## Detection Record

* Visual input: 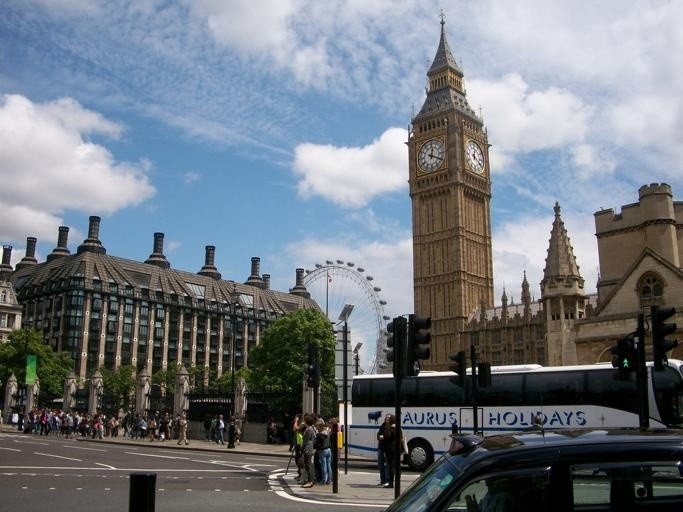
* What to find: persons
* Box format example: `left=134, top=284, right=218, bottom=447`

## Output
left=375, top=414, right=391, bottom=486
left=462, top=475, right=511, bottom=512
left=0, top=406, right=292, bottom=446
left=381, top=415, right=404, bottom=488
left=290, top=412, right=343, bottom=488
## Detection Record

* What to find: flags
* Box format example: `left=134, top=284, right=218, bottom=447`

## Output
left=24, top=355, right=37, bottom=384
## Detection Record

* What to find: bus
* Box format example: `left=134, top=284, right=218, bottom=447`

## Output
left=349, top=359, right=683, bottom=472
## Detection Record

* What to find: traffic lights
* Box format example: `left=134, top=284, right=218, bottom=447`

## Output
left=449, top=351, right=466, bottom=389
left=610, top=337, right=636, bottom=381
left=387, top=314, right=432, bottom=380
left=650, top=305, right=679, bottom=372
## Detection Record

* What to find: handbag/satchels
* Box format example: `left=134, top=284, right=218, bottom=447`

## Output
left=297, top=433, right=302, bottom=445
left=402, top=437, right=408, bottom=454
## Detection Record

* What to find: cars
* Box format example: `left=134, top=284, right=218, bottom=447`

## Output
left=383, top=428, right=683, bottom=512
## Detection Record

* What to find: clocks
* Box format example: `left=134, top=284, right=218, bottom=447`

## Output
left=416, top=138, right=445, bottom=173
left=464, top=139, right=485, bottom=175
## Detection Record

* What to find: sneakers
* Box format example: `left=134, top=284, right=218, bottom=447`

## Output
left=376, top=483, right=393, bottom=488
left=295, top=476, right=331, bottom=488
